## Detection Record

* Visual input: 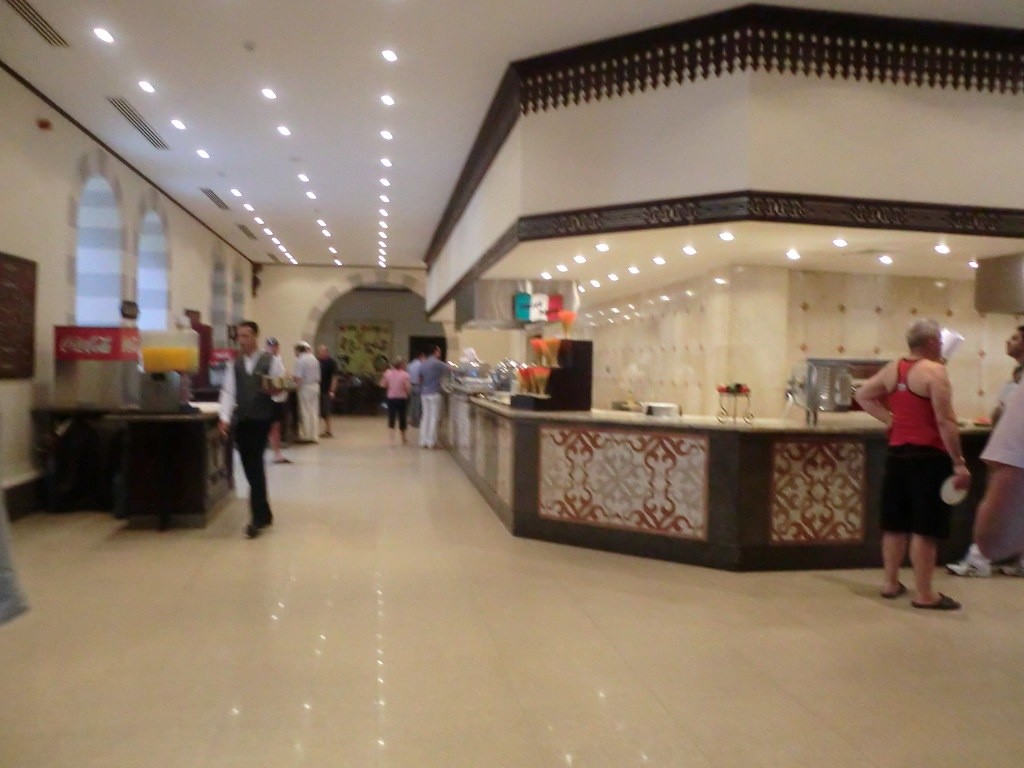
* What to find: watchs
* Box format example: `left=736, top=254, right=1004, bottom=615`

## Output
left=329, top=387, right=336, bottom=393
left=959, top=457, right=966, bottom=464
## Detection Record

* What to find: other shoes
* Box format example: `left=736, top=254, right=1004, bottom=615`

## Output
left=272, top=458, right=290, bottom=464
left=946, top=556, right=990, bottom=578
left=246, top=512, right=273, bottom=536
left=1001, top=556, right=1023, bottom=576
left=319, top=431, right=332, bottom=437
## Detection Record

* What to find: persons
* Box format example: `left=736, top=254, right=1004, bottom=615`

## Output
left=407, top=353, right=426, bottom=426
left=379, top=356, right=412, bottom=446
left=853, top=319, right=972, bottom=609
left=293, top=341, right=320, bottom=443
left=218, top=322, right=282, bottom=538
left=263, top=337, right=288, bottom=465
left=419, top=344, right=449, bottom=449
left=317, top=344, right=337, bottom=439
left=945, top=326, right=1024, bottom=577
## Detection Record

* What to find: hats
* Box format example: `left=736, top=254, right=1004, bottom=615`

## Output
left=297, top=340, right=311, bottom=349
left=940, top=328, right=966, bottom=360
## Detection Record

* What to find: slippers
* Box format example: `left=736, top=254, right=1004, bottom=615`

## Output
left=882, top=581, right=906, bottom=598
left=911, top=591, right=962, bottom=610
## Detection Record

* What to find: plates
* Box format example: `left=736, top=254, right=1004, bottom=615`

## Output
left=940, top=475, right=968, bottom=505
